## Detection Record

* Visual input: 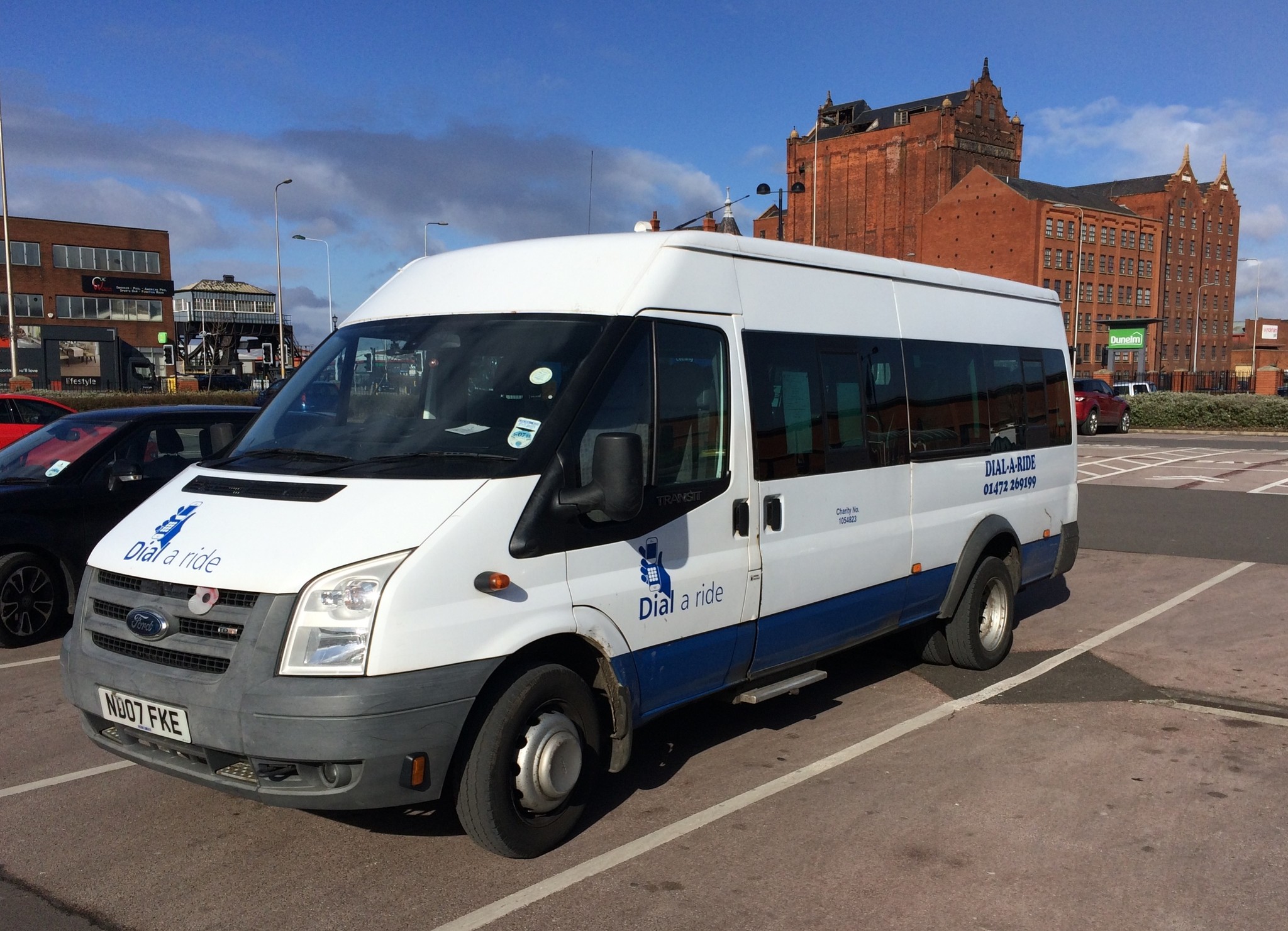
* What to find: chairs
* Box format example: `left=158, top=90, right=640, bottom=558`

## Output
left=579, top=381, right=649, bottom=487
left=59, top=350, right=69, bottom=367
left=84, top=345, right=96, bottom=364
left=144, top=426, right=193, bottom=480
left=658, top=359, right=719, bottom=482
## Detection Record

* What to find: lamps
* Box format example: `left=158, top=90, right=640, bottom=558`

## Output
left=48, top=313, right=53, bottom=318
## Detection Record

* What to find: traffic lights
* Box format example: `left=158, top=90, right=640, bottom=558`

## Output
left=262, top=343, right=273, bottom=364
left=277, top=344, right=288, bottom=364
left=163, top=344, right=175, bottom=366
left=364, top=353, right=373, bottom=373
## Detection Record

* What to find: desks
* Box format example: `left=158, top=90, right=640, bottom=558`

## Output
left=59, top=344, right=89, bottom=367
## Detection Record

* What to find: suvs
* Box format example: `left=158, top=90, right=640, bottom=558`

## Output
left=193, top=373, right=250, bottom=393
left=1113, top=381, right=1157, bottom=398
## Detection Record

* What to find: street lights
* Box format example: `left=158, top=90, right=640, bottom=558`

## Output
left=1193, top=283, right=1216, bottom=373
left=424, top=221, right=449, bottom=256
left=810, top=116, right=835, bottom=246
left=1238, top=258, right=1260, bottom=375
left=756, top=182, right=805, bottom=241
left=1052, top=203, right=1084, bottom=379
left=274, top=178, right=293, bottom=375
left=291, top=235, right=338, bottom=381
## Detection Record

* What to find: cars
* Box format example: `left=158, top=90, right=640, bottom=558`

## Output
left=1237, top=381, right=1248, bottom=391
left=1073, top=377, right=1132, bottom=436
left=0, top=392, right=160, bottom=472
left=253, top=376, right=343, bottom=414
left=362, top=377, right=395, bottom=392
left=0, top=403, right=334, bottom=649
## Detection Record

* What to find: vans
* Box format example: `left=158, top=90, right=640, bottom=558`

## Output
left=61, top=221, right=1079, bottom=860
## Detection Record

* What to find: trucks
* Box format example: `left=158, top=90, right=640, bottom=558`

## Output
left=0, top=325, right=163, bottom=392
